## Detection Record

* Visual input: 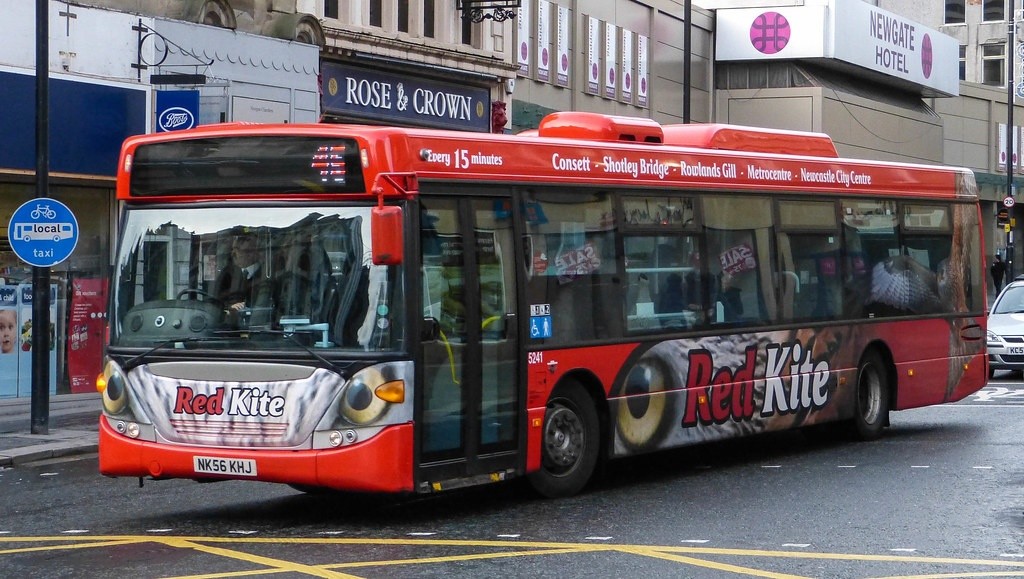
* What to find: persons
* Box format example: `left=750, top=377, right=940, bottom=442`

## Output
left=0, top=311, right=16, bottom=353
left=230, top=232, right=277, bottom=336
left=991, top=255, right=1005, bottom=298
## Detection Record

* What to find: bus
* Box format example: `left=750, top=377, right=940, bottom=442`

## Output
left=94, top=111, right=991, bottom=499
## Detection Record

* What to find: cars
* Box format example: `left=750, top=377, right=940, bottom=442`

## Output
left=987, top=274, right=1024, bottom=380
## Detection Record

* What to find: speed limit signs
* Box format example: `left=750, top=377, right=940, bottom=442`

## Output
left=1003, top=196, right=1015, bottom=208
